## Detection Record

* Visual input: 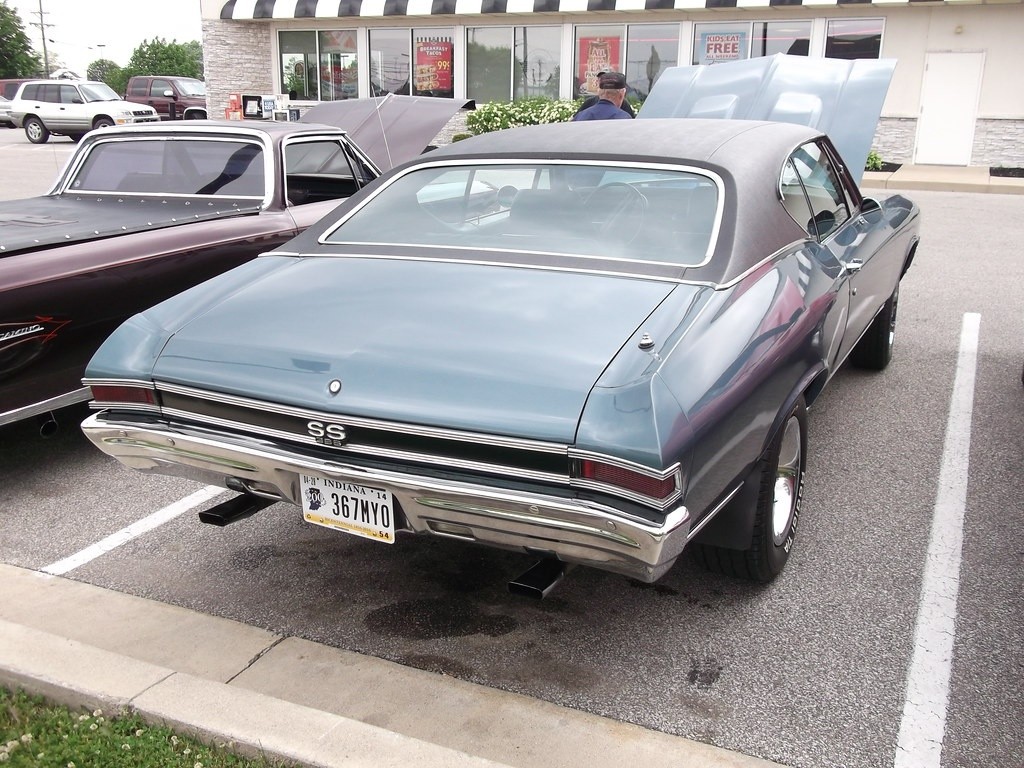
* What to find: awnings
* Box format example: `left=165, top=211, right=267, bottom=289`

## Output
left=220, top=0, right=946, bottom=19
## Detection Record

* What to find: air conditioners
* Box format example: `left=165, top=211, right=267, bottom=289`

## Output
left=273, top=109, right=300, bottom=123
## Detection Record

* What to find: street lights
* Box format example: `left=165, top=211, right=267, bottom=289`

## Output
left=96, top=44, right=106, bottom=59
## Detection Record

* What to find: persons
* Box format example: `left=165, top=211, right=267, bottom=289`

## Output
left=570, top=71, right=635, bottom=121
left=567, top=73, right=633, bottom=186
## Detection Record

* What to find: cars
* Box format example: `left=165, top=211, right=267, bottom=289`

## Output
left=0, top=92, right=496, bottom=439
left=80, top=54, right=923, bottom=606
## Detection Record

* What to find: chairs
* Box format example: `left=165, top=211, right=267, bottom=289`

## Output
left=509, top=188, right=583, bottom=234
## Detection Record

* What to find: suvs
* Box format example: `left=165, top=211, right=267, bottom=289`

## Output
left=125, top=75, right=208, bottom=120
left=6, top=78, right=161, bottom=145
left=0, top=78, right=47, bottom=129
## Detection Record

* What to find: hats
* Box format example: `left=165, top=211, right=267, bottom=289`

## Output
left=599, top=72, right=628, bottom=89
